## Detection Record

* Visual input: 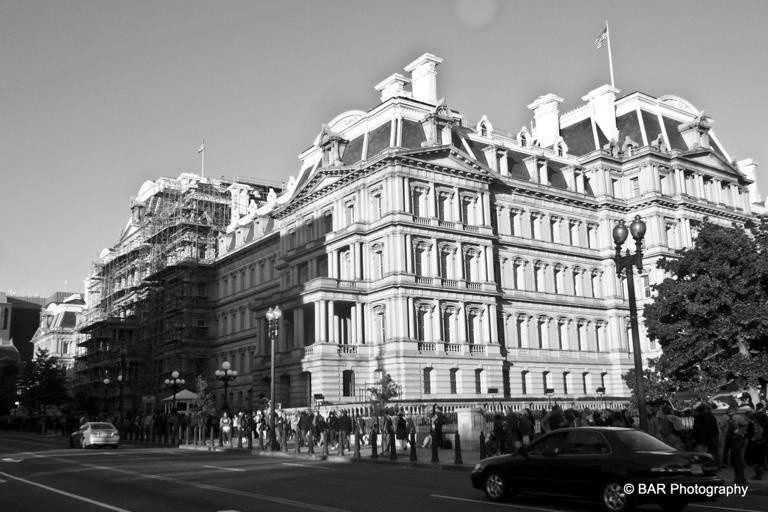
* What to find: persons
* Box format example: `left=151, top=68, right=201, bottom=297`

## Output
left=98, top=396, right=768, bottom=490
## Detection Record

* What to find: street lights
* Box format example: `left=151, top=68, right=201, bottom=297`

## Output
left=264, top=304, right=283, bottom=450
left=611, top=213, right=649, bottom=436
left=214, top=359, right=237, bottom=409
left=163, top=369, right=188, bottom=414
left=115, top=374, right=125, bottom=415
left=102, top=377, right=112, bottom=414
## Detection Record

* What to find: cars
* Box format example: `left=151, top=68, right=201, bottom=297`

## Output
left=70, top=421, right=120, bottom=449
left=467, top=425, right=725, bottom=512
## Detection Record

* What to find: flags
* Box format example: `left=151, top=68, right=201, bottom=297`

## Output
left=593, top=27, right=608, bottom=51
